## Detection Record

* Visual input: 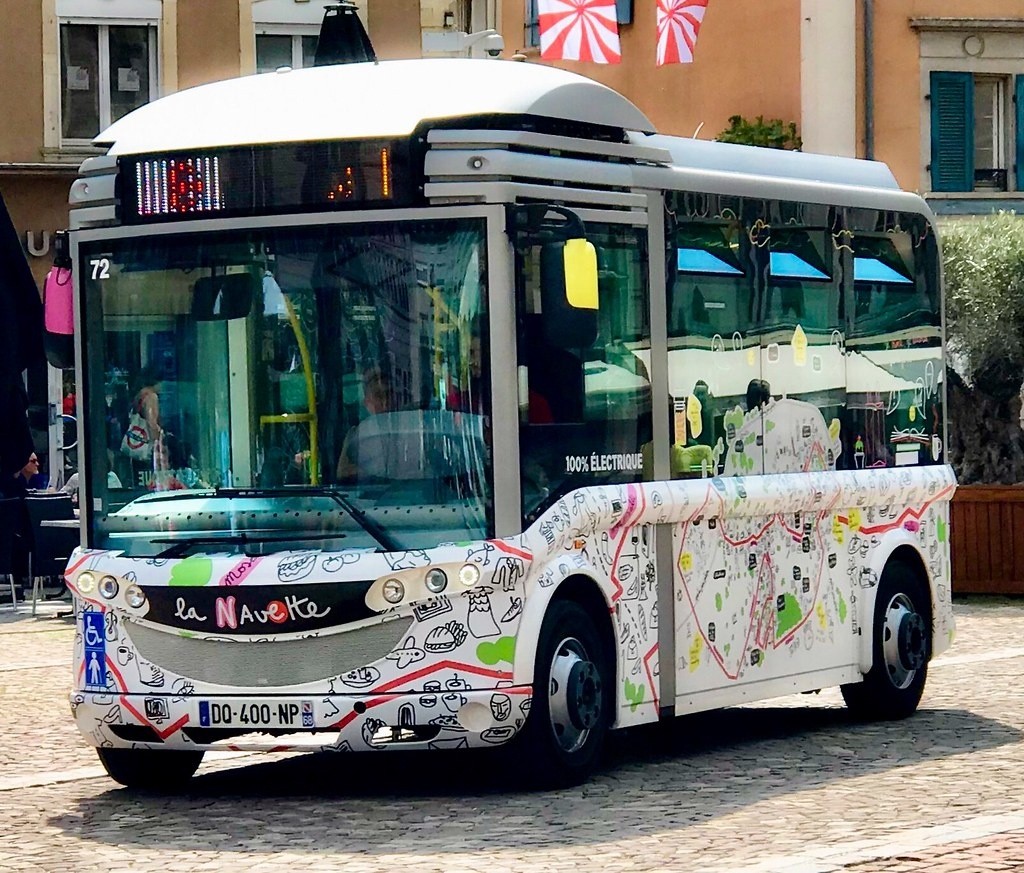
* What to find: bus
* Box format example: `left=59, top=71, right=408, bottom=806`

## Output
left=43, top=57, right=958, bottom=793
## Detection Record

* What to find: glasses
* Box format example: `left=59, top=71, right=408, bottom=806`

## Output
left=30, top=459, right=39, bottom=464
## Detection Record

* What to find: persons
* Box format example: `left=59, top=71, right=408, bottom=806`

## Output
left=128, top=368, right=165, bottom=487
left=0, top=446, right=122, bottom=503
left=358, top=366, right=387, bottom=423
left=445, top=336, right=550, bottom=424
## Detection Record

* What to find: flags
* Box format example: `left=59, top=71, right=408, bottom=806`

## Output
left=538, top=0, right=621, bottom=64
left=656, top=0, right=708, bottom=67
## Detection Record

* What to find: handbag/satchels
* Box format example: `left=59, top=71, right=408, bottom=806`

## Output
left=119, top=390, right=162, bottom=466
